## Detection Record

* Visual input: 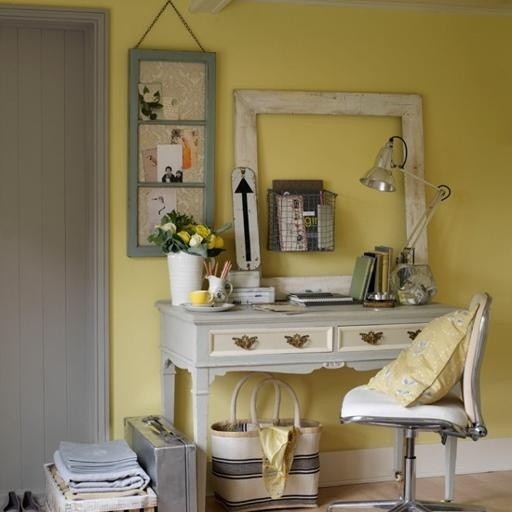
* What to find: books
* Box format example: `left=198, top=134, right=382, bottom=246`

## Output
left=289, top=245, right=394, bottom=306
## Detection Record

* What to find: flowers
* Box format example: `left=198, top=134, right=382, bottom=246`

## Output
left=147, top=206, right=226, bottom=262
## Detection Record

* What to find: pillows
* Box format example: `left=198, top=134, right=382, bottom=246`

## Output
left=367, top=306, right=480, bottom=409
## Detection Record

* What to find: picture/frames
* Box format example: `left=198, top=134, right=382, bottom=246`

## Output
left=233, top=87, right=432, bottom=303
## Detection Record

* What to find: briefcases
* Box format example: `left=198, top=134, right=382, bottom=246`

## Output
left=123, top=415, right=197, bottom=511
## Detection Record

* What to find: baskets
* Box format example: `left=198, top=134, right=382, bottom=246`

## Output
left=267, top=188, right=337, bottom=254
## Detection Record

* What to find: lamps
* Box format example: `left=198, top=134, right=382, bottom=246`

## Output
left=359, top=134, right=452, bottom=308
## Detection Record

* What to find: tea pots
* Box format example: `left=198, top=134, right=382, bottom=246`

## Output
left=205, top=274, right=233, bottom=304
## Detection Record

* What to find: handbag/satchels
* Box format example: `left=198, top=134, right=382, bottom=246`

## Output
left=210, top=373, right=322, bottom=512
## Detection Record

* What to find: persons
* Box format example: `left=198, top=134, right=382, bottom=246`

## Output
left=175, top=170, right=182, bottom=183
left=162, top=166, right=175, bottom=183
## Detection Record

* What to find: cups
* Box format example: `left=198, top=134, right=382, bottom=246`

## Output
left=188, top=289, right=214, bottom=304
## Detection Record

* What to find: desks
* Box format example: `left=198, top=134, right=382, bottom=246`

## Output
left=155, top=296, right=458, bottom=511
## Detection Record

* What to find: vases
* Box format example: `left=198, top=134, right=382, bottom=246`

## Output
left=166, top=252, right=206, bottom=306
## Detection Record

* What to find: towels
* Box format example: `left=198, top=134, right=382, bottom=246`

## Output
left=46, top=439, right=152, bottom=502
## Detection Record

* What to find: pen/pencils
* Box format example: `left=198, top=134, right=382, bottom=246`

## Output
left=203, top=261, right=233, bottom=279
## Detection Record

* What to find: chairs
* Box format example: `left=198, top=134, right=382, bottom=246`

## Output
left=328, top=291, right=493, bottom=512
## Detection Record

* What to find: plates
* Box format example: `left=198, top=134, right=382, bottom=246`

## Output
left=179, top=301, right=234, bottom=312
